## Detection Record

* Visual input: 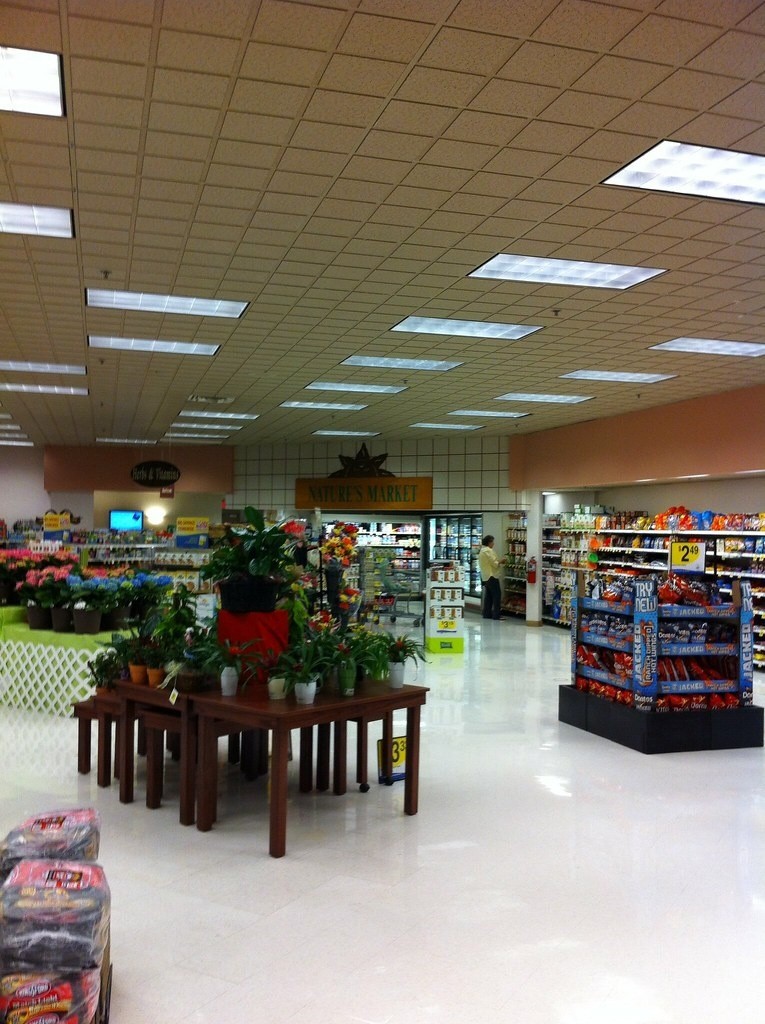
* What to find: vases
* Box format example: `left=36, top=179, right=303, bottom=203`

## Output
left=130, top=600, right=160, bottom=629
left=51, top=607, right=74, bottom=633
left=100, top=606, right=131, bottom=631
left=322, top=569, right=344, bottom=625
left=72, top=609, right=101, bottom=634
left=301, top=597, right=317, bottom=616
left=27, top=606, right=52, bottom=630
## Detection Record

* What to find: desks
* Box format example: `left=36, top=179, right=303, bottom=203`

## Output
left=113, top=677, right=329, bottom=824
left=191, top=682, right=431, bottom=859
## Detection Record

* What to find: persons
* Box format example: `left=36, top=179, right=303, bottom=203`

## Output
left=478, top=535, right=508, bottom=620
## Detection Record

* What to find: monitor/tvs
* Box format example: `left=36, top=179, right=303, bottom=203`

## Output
left=108, top=510, right=144, bottom=533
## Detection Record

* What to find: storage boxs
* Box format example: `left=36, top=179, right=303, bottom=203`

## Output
left=186, top=549, right=199, bottom=568
left=430, top=589, right=441, bottom=600
left=171, top=550, right=182, bottom=565
left=199, top=572, right=210, bottom=591
left=177, top=549, right=187, bottom=565
left=186, top=572, right=199, bottom=592
left=452, top=589, right=462, bottom=599
left=175, top=571, right=186, bottom=591
left=162, top=551, right=171, bottom=564
left=452, top=608, right=462, bottom=618
left=199, top=549, right=213, bottom=565
left=455, top=571, right=463, bottom=581
left=442, top=607, right=452, bottom=618
left=431, top=571, right=444, bottom=581
left=154, top=552, right=164, bottom=563
left=431, top=607, right=442, bottom=617
left=174, top=517, right=208, bottom=550
left=444, top=571, right=455, bottom=581
left=441, top=589, right=452, bottom=599
left=43, top=514, right=71, bottom=543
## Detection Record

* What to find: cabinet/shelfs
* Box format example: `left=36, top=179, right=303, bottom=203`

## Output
left=542, top=503, right=765, bottom=673
left=501, top=514, right=527, bottom=617
left=0, top=529, right=217, bottom=621
left=425, top=559, right=464, bottom=654
left=559, top=569, right=765, bottom=756
left=345, top=520, right=422, bottom=594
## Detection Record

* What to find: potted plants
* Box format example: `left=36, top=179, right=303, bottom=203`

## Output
left=381, top=631, right=429, bottom=689
left=282, top=641, right=332, bottom=705
left=254, top=650, right=291, bottom=700
left=88, top=656, right=115, bottom=697
left=143, top=641, right=172, bottom=687
left=328, top=630, right=376, bottom=697
left=199, top=639, right=264, bottom=698
left=197, top=506, right=304, bottom=682
left=332, top=635, right=378, bottom=687
left=123, top=636, right=147, bottom=685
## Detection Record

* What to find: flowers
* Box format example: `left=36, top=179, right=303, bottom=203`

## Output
left=229, top=647, right=243, bottom=668
left=133, top=573, right=175, bottom=600
left=15, top=569, right=56, bottom=607
left=37, top=567, right=74, bottom=609
left=317, top=522, right=359, bottom=571
left=395, top=640, right=407, bottom=650
left=337, top=584, right=363, bottom=626
left=301, top=571, right=321, bottom=599
left=0, top=547, right=82, bottom=580
left=66, top=575, right=114, bottom=613
left=307, top=611, right=341, bottom=638
left=101, top=572, right=142, bottom=607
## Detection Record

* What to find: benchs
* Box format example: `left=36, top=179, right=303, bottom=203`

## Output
left=143, top=709, right=276, bottom=827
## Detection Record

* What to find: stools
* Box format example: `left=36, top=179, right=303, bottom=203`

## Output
left=73, top=700, right=146, bottom=786
left=346, top=710, right=393, bottom=793
